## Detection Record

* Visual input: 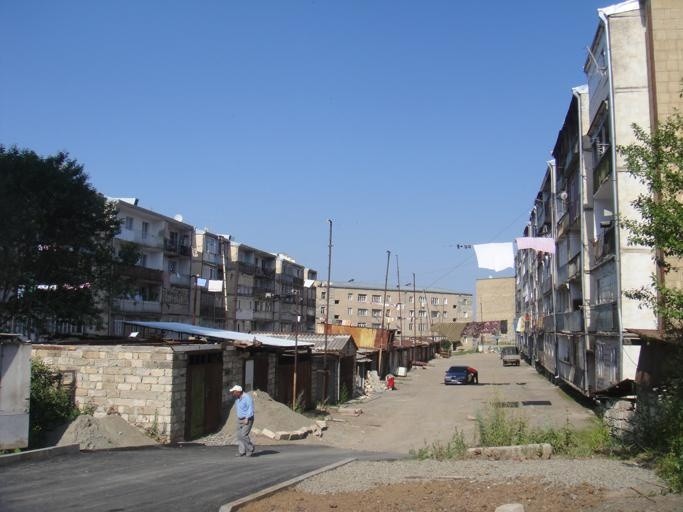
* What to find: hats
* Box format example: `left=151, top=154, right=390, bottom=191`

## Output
left=229, top=385, right=242, bottom=392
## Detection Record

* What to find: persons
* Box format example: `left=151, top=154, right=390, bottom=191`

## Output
left=229, top=385, right=255, bottom=456
left=386, top=373, right=394, bottom=390
left=467, top=367, right=478, bottom=384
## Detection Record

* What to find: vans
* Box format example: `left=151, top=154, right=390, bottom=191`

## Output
left=501, top=345, right=521, bottom=366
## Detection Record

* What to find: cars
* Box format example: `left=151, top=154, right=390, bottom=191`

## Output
left=444, top=366, right=478, bottom=385
